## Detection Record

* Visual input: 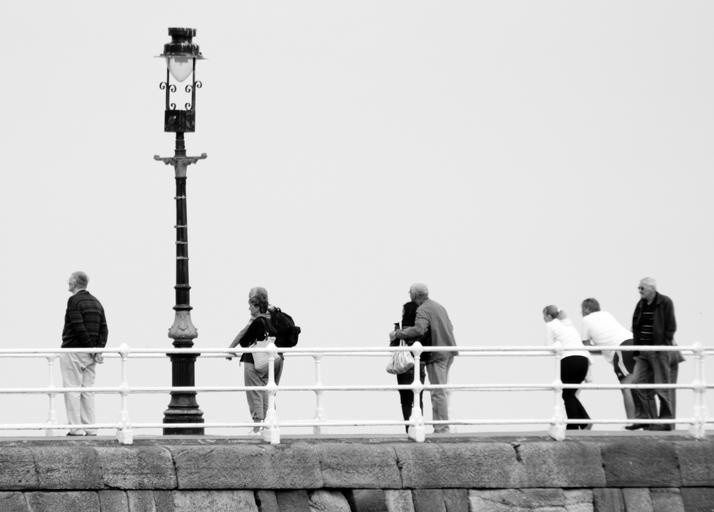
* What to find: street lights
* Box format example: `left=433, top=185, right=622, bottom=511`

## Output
left=150, top=24, right=214, bottom=439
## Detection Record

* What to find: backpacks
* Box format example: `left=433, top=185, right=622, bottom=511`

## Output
left=266, top=306, right=301, bottom=348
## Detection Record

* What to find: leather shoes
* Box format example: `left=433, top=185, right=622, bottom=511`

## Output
left=624, top=422, right=650, bottom=431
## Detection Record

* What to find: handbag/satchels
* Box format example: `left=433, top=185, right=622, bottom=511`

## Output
left=248, top=335, right=282, bottom=373
left=668, top=344, right=686, bottom=367
left=385, top=337, right=415, bottom=375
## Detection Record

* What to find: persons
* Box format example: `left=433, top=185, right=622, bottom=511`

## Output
left=58, top=271, right=108, bottom=437
left=240, top=292, right=273, bottom=434
left=625, top=277, right=684, bottom=431
left=580, top=299, right=644, bottom=430
left=390, top=283, right=459, bottom=435
left=226, top=288, right=285, bottom=387
left=542, top=306, right=593, bottom=430
